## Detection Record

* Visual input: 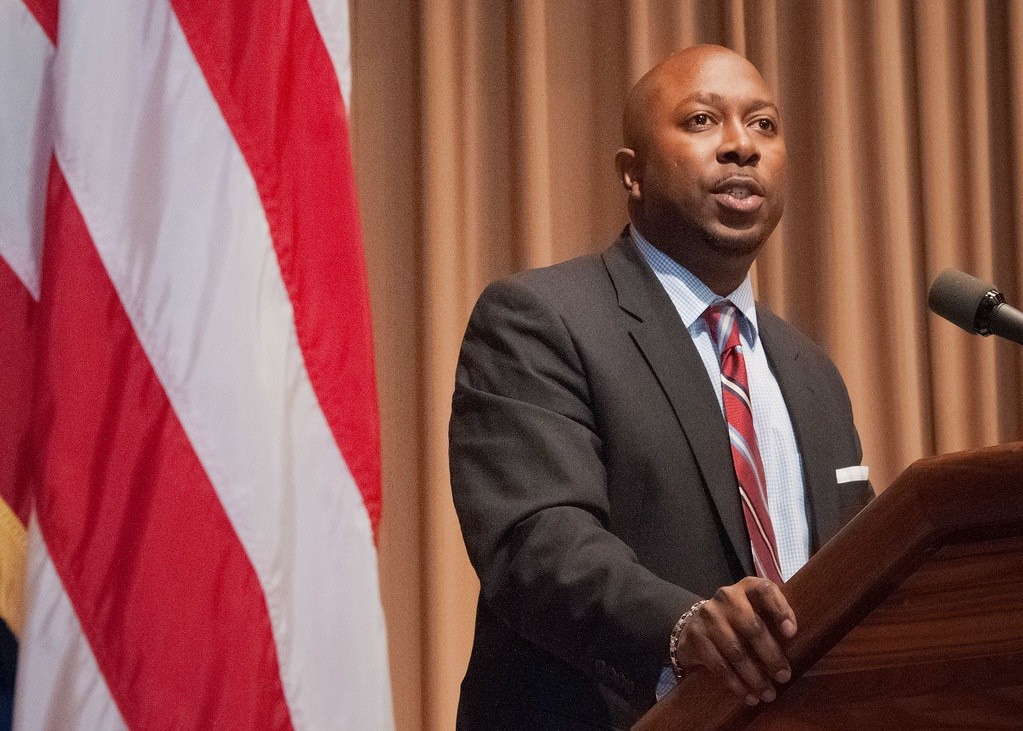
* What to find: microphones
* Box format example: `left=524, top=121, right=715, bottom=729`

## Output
left=928, top=268, right=1023, bottom=344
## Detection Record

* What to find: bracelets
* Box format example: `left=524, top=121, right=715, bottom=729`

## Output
left=670, top=600, right=709, bottom=682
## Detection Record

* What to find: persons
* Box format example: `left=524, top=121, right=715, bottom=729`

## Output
left=449, top=45, right=877, bottom=731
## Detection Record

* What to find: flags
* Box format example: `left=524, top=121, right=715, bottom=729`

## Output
left=1, top=2, right=395, bottom=730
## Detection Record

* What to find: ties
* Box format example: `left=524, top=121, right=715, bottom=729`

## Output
left=699, top=299, right=784, bottom=591
